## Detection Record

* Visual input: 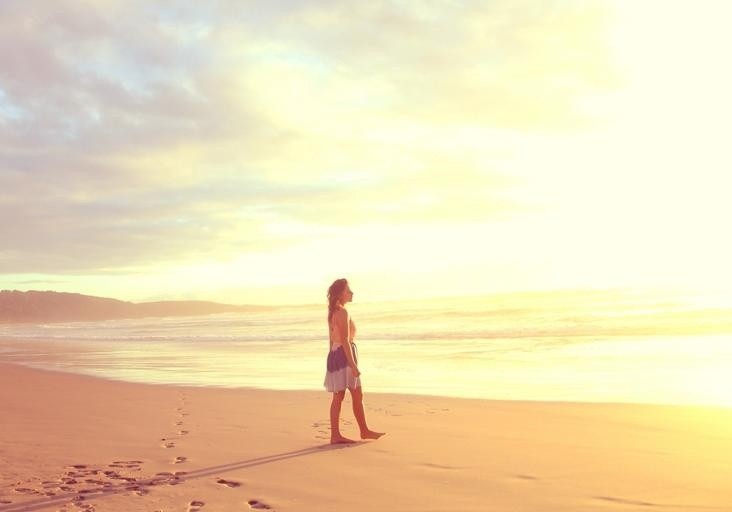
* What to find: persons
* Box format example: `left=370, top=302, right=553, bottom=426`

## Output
left=322, top=277, right=386, bottom=444
